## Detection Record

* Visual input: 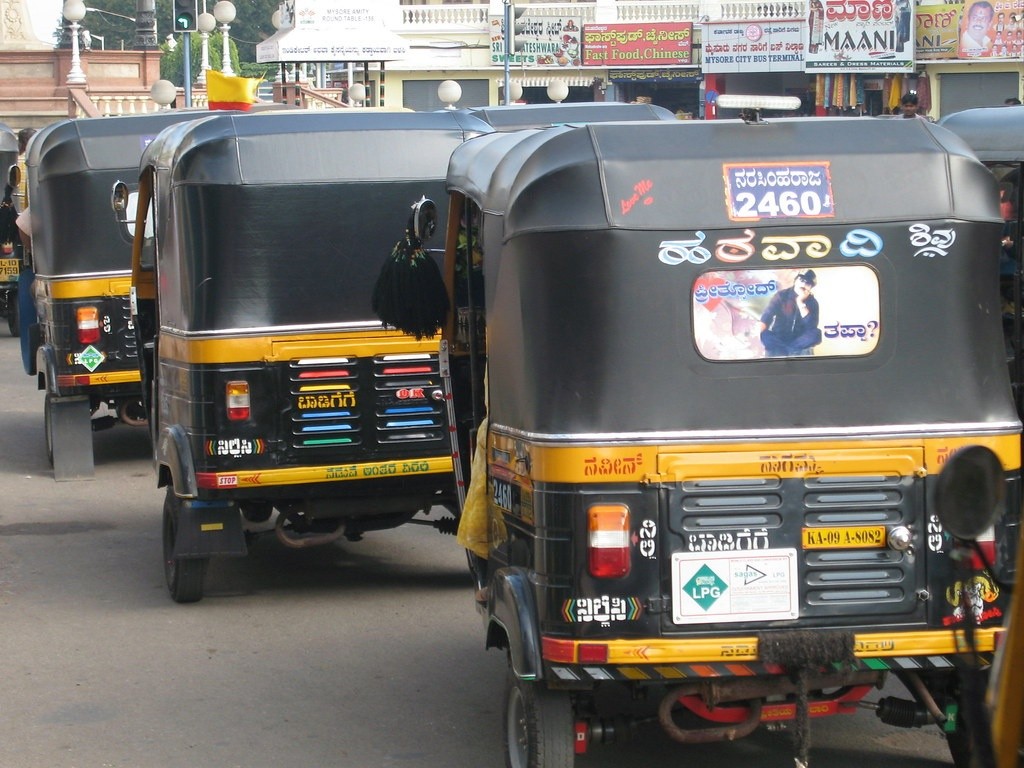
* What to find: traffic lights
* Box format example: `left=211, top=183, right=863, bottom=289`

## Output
left=173, top=0, right=199, bottom=33
left=509, top=4, right=527, bottom=56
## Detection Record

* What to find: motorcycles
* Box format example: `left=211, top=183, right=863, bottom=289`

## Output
left=452, top=102, right=680, bottom=132
left=110, top=111, right=498, bottom=603
left=407, top=94, right=1022, bottom=767
left=934, top=444, right=1024, bottom=768
left=933, top=106, right=1023, bottom=409
left=6, top=110, right=250, bottom=482
left=0, top=127, right=26, bottom=332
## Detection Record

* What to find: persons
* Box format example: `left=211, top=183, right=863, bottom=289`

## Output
left=15, top=205, right=34, bottom=253
left=897, top=92, right=929, bottom=122
left=751, top=270, right=825, bottom=359
left=960, top=1, right=993, bottom=55
left=990, top=11, right=1024, bottom=58
left=1005, top=97, right=1022, bottom=106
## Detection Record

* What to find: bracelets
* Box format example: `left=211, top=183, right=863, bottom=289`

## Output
left=798, top=303, right=807, bottom=309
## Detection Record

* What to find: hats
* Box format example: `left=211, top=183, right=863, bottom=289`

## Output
left=798, top=269, right=817, bottom=285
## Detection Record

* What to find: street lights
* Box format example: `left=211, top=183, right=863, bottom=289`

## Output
left=214, top=1, right=236, bottom=76
left=151, top=79, right=176, bottom=112
left=437, top=80, right=462, bottom=110
left=197, top=13, right=216, bottom=83
left=350, top=83, right=365, bottom=107
left=62, top=0, right=88, bottom=119
left=271, top=10, right=294, bottom=83
left=85, top=7, right=158, bottom=43
left=82, top=30, right=104, bottom=51
left=503, top=82, right=523, bottom=103
left=547, top=80, right=568, bottom=103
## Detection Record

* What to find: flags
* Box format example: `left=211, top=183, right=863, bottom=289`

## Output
left=204, top=68, right=261, bottom=112
